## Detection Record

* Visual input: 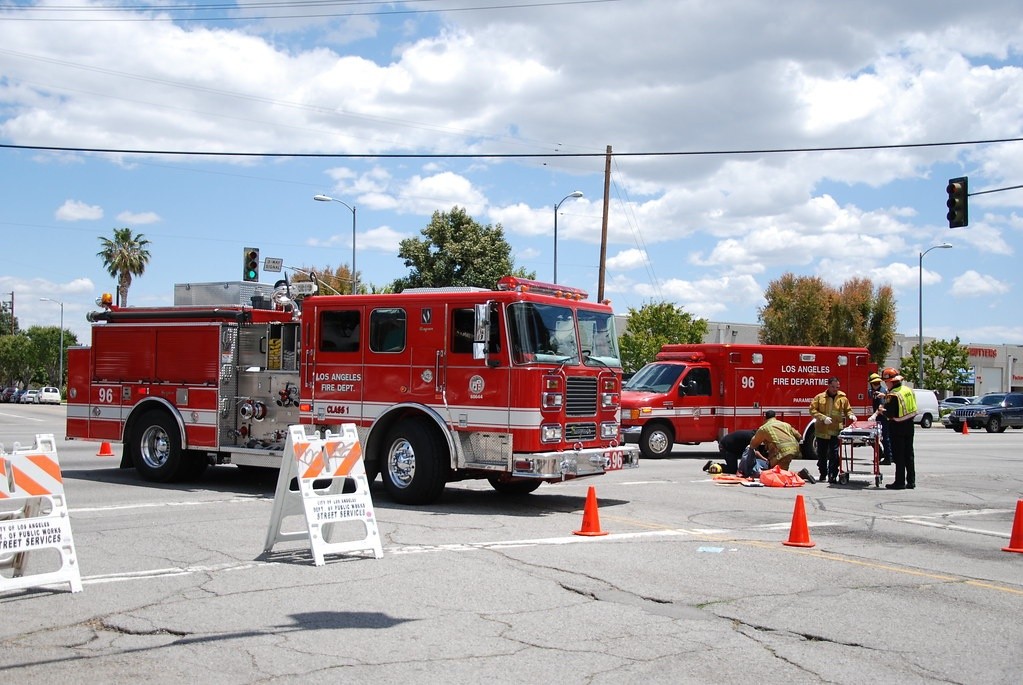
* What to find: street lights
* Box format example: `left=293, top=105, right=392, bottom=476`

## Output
left=554, top=191, right=583, bottom=283
left=314, top=194, right=356, bottom=294
left=40, top=298, right=63, bottom=388
left=919, top=243, right=954, bottom=389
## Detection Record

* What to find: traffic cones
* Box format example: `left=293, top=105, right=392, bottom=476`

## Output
left=572, top=485, right=610, bottom=536
left=96, top=442, right=114, bottom=456
left=783, top=495, right=815, bottom=548
left=1001, top=499, right=1023, bottom=553
left=962, top=421, right=970, bottom=435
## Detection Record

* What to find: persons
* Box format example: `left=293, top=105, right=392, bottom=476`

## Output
left=867, top=368, right=923, bottom=489
left=753, top=411, right=816, bottom=485
left=810, top=377, right=858, bottom=485
left=703, top=427, right=765, bottom=474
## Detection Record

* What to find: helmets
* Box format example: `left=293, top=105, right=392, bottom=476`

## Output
left=881, top=368, right=904, bottom=381
left=868, top=373, right=882, bottom=383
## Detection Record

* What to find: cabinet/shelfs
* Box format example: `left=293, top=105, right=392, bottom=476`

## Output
left=839, top=420, right=884, bottom=486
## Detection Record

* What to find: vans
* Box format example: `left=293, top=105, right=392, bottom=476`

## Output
left=911, top=389, right=939, bottom=427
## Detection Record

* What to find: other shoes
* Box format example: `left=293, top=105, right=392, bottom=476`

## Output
left=906, top=483, right=913, bottom=489
left=886, top=480, right=905, bottom=489
left=820, top=473, right=826, bottom=482
left=799, top=468, right=816, bottom=483
left=828, top=475, right=836, bottom=484
left=878, top=460, right=890, bottom=464
left=702, top=460, right=712, bottom=471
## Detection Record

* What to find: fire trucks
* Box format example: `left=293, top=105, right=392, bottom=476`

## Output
left=620, top=342, right=879, bottom=459
left=64, top=282, right=639, bottom=505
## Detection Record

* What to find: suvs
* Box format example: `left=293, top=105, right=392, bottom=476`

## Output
left=950, top=391, right=1023, bottom=434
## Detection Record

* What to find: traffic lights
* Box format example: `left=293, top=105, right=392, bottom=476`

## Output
left=244, top=247, right=259, bottom=282
left=946, top=176, right=968, bottom=228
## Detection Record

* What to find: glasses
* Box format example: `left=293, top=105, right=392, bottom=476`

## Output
left=872, top=382, right=880, bottom=386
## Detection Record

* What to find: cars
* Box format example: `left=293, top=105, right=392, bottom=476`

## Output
left=0, top=386, right=61, bottom=404
left=937, top=397, right=975, bottom=408
left=942, top=412, right=952, bottom=429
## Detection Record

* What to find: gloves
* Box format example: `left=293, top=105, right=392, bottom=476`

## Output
left=868, top=414, right=878, bottom=422
left=824, top=416, right=833, bottom=425
left=849, top=415, right=857, bottom=421
